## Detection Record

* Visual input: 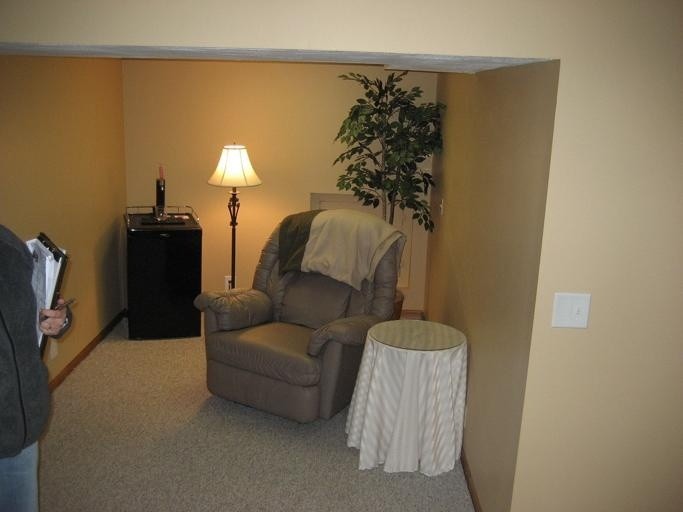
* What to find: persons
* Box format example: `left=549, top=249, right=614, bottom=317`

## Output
left=0, top=223, right=72, bottom=512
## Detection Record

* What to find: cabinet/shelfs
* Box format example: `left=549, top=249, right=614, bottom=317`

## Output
left=127, top=230, right=201, bottom=340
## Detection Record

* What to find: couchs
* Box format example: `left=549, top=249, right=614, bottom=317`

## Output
left=193, top=208, right=407, bottom=424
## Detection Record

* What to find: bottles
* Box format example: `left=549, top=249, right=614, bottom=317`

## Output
left=156, top=166, right=165, bottom=208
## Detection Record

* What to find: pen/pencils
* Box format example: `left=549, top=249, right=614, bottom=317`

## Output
left=37, top=297, right=76, bottom=323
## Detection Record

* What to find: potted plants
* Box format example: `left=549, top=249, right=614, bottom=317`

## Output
left=330, top=70, right=448, bottom=320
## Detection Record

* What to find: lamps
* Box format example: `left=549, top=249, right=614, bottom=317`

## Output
left=207, top=145, right=263, bottom=289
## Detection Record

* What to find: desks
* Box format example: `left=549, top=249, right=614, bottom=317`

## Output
left=345, top=319, right=468, bottom=477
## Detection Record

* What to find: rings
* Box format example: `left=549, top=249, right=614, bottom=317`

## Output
left=49, top=324, right=52, bottom=332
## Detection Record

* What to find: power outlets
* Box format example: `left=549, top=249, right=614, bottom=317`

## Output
left=223, top=276, right=236, bottom=289
